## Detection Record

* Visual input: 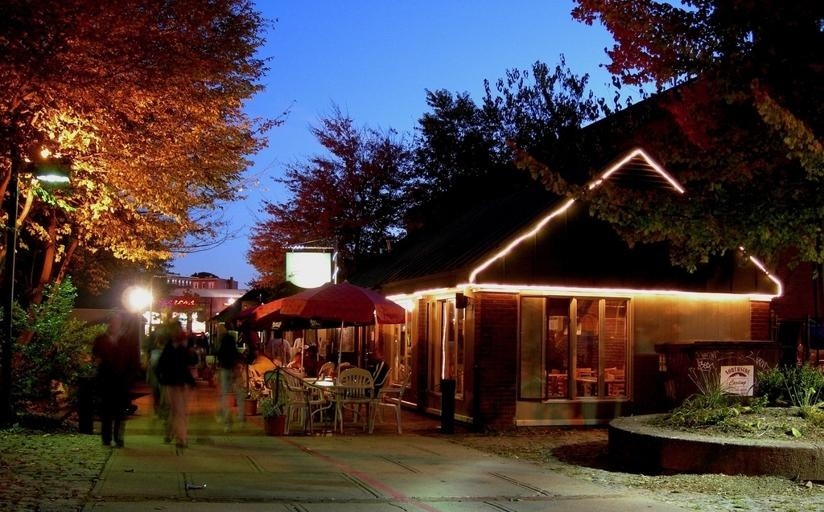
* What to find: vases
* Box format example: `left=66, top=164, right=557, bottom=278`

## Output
left=223, top=392, right=239, bottom=407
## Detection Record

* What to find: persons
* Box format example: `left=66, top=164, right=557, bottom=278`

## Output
left=81, top=313, right=387, bottom=449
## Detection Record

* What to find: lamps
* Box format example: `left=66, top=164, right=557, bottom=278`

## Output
left=455, top=292, right=470, bottom=309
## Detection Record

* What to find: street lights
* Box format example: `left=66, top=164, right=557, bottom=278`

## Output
left=0, top=157, right=73, bottom=431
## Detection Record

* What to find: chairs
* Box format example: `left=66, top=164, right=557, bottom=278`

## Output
left=547, top=367, right=625, bottom=398
left=262, top=337, right=413, bottom=434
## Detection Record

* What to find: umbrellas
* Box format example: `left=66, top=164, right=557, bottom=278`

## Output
left=247, top=286, right=304, bottom=331
left=280, top=277, right=407, bottom=381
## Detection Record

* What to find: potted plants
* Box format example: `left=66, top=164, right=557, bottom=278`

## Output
left=245, top=388, right=286, bottom=435
